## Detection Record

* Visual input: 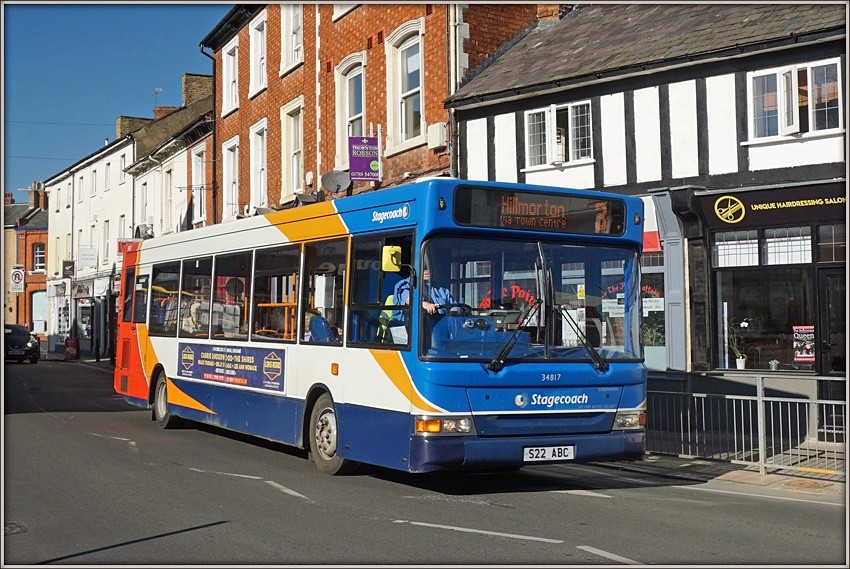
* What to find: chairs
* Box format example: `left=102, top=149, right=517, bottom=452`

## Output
left=123, top=273, right=455, bottom=345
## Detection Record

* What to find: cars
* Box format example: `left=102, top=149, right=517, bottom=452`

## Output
left=4, top=324, right=39, bottom=363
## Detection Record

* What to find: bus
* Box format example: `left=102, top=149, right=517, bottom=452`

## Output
left=113, top=180, right=647, bottom=475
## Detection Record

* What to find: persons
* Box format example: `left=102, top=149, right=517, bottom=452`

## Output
left=305, top=310, right=321, bottom=341
left=184, top=302, right=203, bottom=333
left=393, top=258, right=460, bottom=321
left=240, top=319, right=248, bottom=334
